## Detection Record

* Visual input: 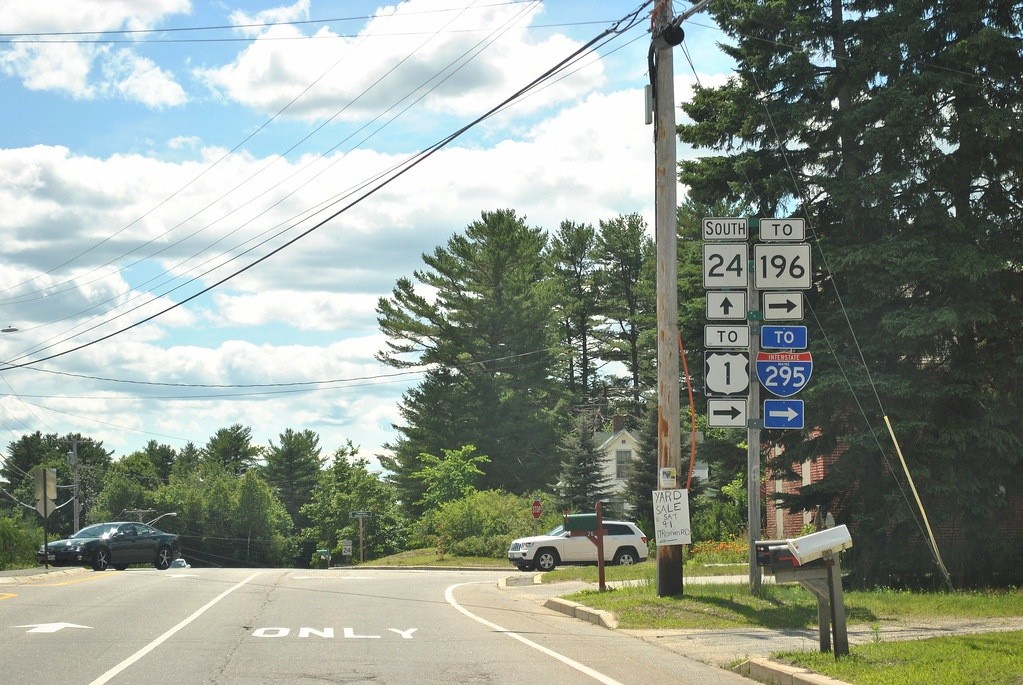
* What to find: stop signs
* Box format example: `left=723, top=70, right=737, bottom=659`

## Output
left=532, top=501, right=542, bottom=519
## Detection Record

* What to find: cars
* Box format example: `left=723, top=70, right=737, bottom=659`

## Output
left=37, top=522, right=182, bottom=571
left=169, top=560, right=191, bottom=569
left=508, top=521, right=649, bottom=572
